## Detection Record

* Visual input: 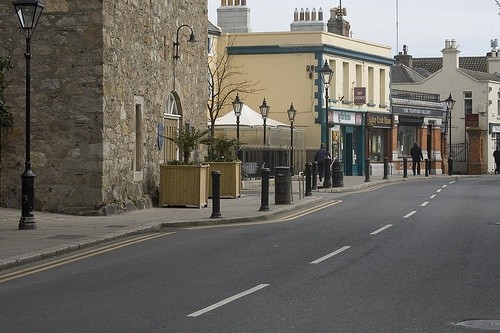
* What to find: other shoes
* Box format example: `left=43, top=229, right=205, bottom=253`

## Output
left=495, top=169, right=496, bottom=174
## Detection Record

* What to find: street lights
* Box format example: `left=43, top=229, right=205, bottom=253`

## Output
left=287, top=102, right=297, bottom=175
left=319, top=59, right=334, bottom=188
left=12, top=0, right=46, bottom=231
left=445, top=92, right=456, bottom=175
left=259, top=97, right=270, bottom=167
left=232, top=92, right=244, bottom=158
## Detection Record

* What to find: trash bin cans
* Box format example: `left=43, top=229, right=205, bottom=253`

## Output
left=274, top=166, right=291, bottom=205
left=333, top=161, right=343, bottom=187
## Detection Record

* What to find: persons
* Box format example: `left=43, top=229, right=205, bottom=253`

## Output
left=313, top=143, right=330, bottom=185
left=410, top=141, right=424, bottom=176
left=493, top=146, right=500, bottom=175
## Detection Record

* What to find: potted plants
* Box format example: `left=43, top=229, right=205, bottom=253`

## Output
left=204, top=133, right=247, bottom=199
left=155, top=120, right=218, bottom=209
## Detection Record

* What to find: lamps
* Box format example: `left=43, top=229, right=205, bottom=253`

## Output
left=173, top=23, right=200, bottom=63
